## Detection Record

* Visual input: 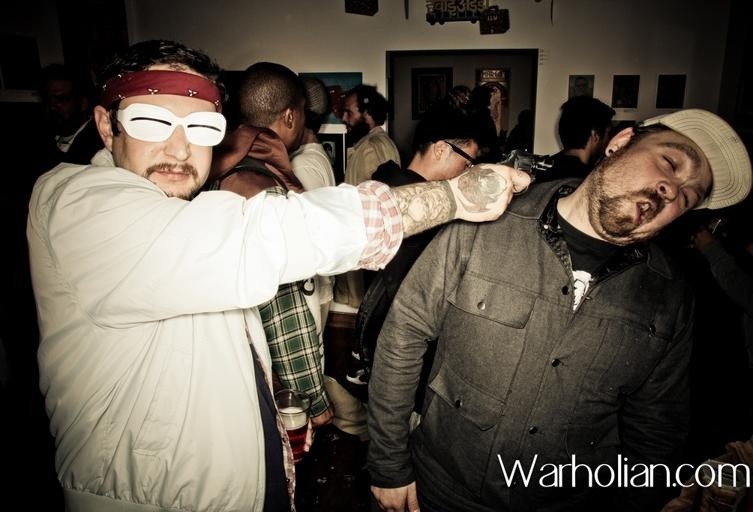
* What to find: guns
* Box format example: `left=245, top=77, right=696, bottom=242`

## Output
left=496, top=150, right=554, bottom=195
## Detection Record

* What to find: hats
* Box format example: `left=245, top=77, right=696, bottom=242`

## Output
left=640, top=108, right=753, bottom=211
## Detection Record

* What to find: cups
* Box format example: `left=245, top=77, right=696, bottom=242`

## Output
left=273, top=388, right=310, bottom=464
